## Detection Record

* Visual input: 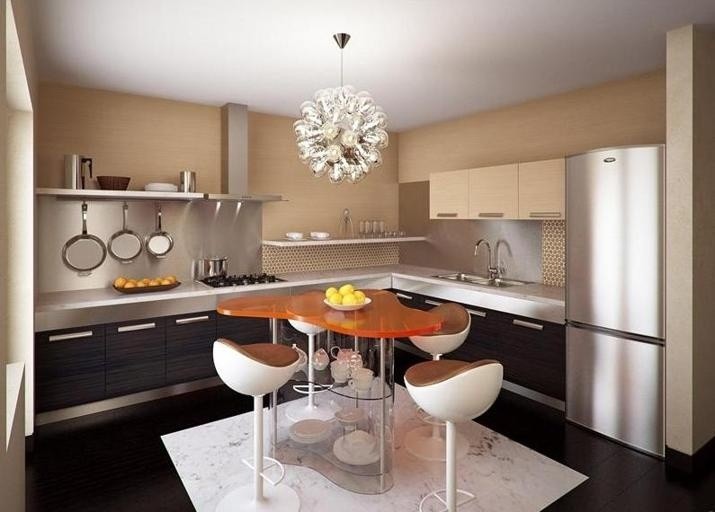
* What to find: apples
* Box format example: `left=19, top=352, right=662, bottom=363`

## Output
left=113, top=275, right=175, bottom=288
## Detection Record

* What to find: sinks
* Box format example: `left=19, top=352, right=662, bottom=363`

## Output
left=429, top=272, right=488, bottom=283
left=473, top=277, right=537, bottom=288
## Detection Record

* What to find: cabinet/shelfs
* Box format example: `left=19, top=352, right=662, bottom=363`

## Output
left=518, top=158, right=566, bottom=220
left=216, top=310, right=270, bottom=386
left=392, top=288, right=496, bottom=361
left=429, top=170, right=470, bottom=221
left=469, top=163, right=518, bottom=220
left=496, top=311, right=565, bottom=413
left=36, top=324, right=107, bottom=427
left=106, top=310, right=217, bottom=410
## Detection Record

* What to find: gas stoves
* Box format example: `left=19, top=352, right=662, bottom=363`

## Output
left=198, top=271, right=288, bottom=289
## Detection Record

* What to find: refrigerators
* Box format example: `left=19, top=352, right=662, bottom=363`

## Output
left=561, top=143, right=668, bottom=463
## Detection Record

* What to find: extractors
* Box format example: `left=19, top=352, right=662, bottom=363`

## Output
left=204, top=103, right=286, bottom=203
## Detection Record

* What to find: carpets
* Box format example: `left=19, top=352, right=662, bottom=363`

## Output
left=160, top=378, right=589, bottom=512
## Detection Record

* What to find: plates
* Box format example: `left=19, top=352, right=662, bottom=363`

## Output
left=282, top=238, right=306, bottom=241
left=111, top=281, right=181, bottom=293
left=307, top=237, right=332, bottom=241
left=323, top=296, right=372, bottom=312
left=289, top=407, right=381, bottom=465
left=144, top=183, right=178, bottom=193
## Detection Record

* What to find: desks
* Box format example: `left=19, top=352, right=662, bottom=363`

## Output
left=215, top=289, right=443, bottom=492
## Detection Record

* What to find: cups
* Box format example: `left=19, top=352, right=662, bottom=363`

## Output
left=63, top=154, right=93, bottom=189
left=285, top=232, right=303, bottom=238
left=310, top=232, right=329, bottom=237
left=313, top=346, right=378, bottom=393
left=359, top=215, right=408, bottom=238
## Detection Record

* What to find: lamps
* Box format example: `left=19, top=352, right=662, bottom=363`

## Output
left=292, top=33, right=390, bottom=184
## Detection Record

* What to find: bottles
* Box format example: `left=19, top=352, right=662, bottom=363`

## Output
left=179, top=168, right=197, bottom=192
left=337, top=209, right=356, bottom=239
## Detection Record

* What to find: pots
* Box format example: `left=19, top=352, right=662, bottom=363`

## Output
left=60, top=201, right=173, bottom=275
left=197, top=257, right=232, bottom=278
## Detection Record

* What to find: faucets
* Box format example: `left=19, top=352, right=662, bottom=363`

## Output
left=473, top=239, right=497, bottom=279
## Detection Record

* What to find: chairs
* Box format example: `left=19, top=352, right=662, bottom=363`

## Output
left=401, top=303, right=472, bottom=464
left=404, top=360, right=504, bottom=512
left=286, top=291, right=334, bottom=445
left=212, top=337, right=301, bottom=512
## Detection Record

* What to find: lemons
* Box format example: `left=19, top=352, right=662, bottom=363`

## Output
left=325, top=284, right=365, bottom=306
